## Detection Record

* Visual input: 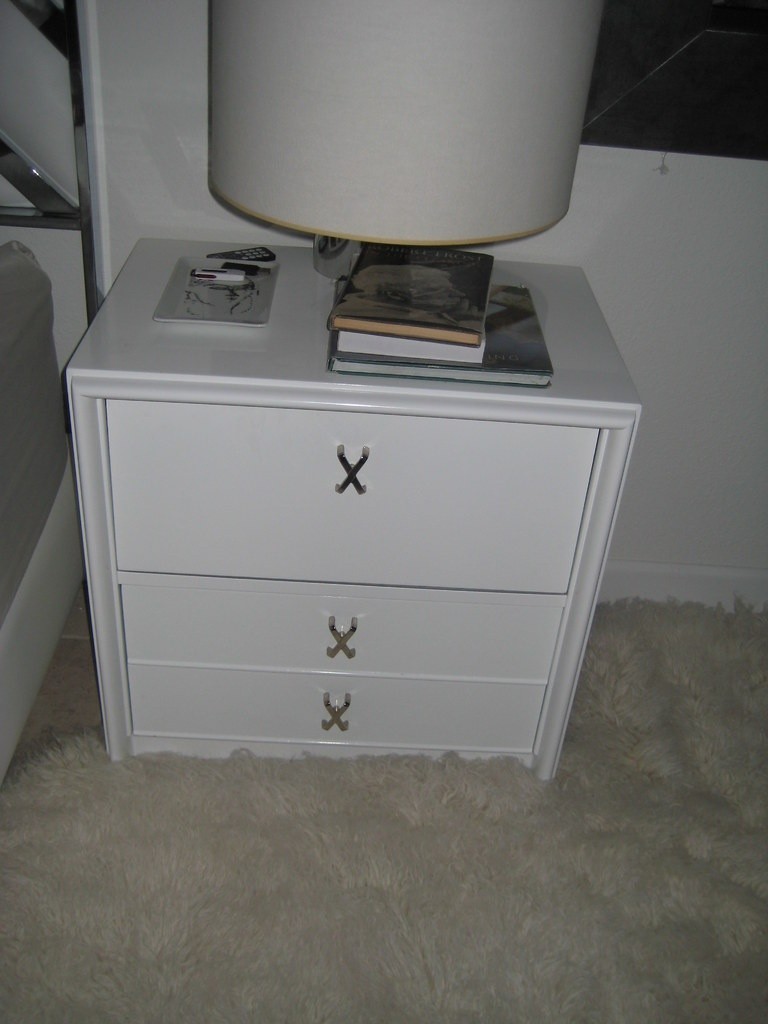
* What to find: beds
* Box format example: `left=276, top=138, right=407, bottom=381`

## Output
left=0, top=0, right=123, bottom=789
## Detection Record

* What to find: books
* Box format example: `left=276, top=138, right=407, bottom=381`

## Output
left=326, top=243, right=494, bottom=348
left=326, top=284, right=554, bottom=389
left=337, top=331, right=486, bottom=363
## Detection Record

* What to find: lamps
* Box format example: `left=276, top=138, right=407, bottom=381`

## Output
left=204, top=0, right=604, bottom=275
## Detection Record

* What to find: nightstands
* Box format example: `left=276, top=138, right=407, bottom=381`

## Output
left=64, top=237, right=643, bottom=778
